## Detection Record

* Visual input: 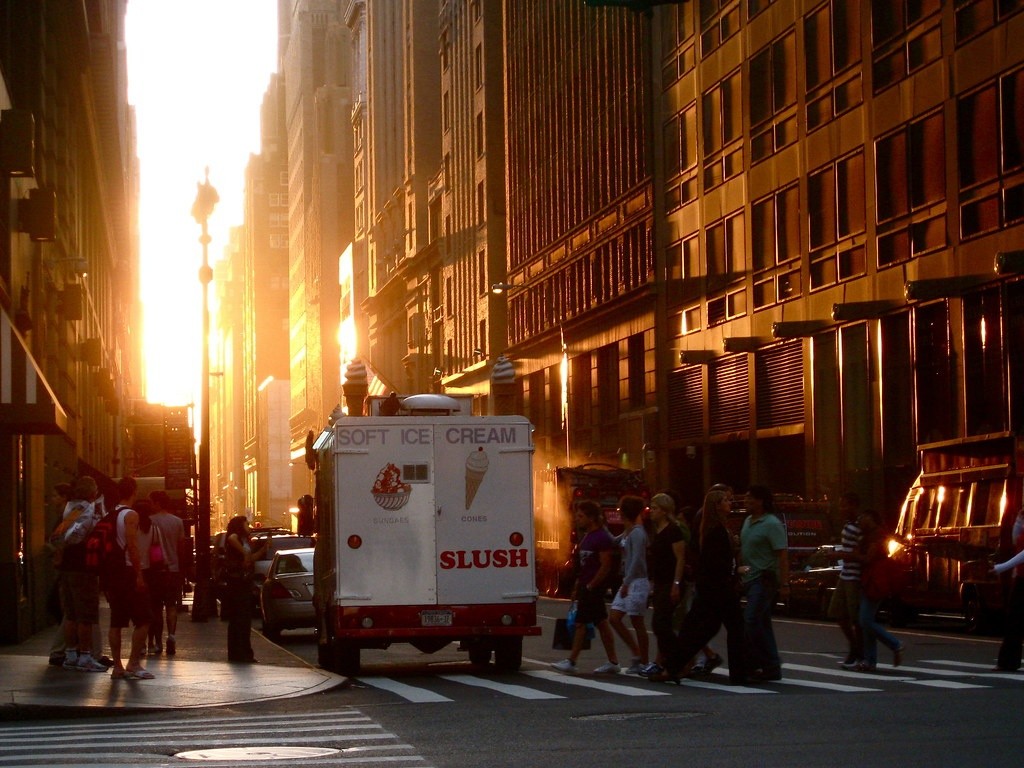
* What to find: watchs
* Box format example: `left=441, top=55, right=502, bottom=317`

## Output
left=673, top=580, right=680, bottom=584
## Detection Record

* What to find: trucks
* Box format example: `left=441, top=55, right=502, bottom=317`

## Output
left=295, top=394, right=542, bottom=677
left=877, top=431, right=1024, bottom=634
left=533, top=463, right=649, bottom=598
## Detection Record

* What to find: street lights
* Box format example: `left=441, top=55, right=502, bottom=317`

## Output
left=191, top=164, right=221, bottom=620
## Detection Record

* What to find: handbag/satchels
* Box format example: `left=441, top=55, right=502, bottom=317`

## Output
left=225, top=567, right=247, bottom=584
left=51, top=499, right=95, bottom=546
left=150, top=522, right=165, bottom=572
left=554, top=598, right=594, bottom=650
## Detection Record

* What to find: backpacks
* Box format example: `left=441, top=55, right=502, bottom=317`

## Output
left=82, top=506, right=138, bottom=575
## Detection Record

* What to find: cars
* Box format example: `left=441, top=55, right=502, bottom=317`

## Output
left=210, top=527, right=317, bottom=611
left=259, top=547, right=316, bottom=641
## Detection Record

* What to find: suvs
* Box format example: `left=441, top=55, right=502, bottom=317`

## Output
left=687, top=492, right=834, bottom=615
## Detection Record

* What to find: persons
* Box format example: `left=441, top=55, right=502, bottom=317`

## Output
left=824, top=498, right=907, bottom=672
left=552, top=500, right=621, bottom=674
left=44, top=475, right=168, bottom=680
left=147, top=490, right=184, bottom=653
left=608, top=484, right=754, bottom=685
left=741, top=486, right=789, bottom=682
left=221, top=515, right=274, bottom=664
left=987, top=506, right=1024, bottom=674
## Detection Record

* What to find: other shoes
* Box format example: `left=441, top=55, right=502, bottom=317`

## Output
left=849, top=661, right=875, bottom=672
left=553, top=657, right=781, bottom=683
left=49, top=637, right=175, bottom=680
left=894, top=641, right=906, bottom=666
left=841, top=660, right=860, bottom=669
left=993, top=663, right=1020, bottom=672
left=231, top=654, right=260, bottom=664
